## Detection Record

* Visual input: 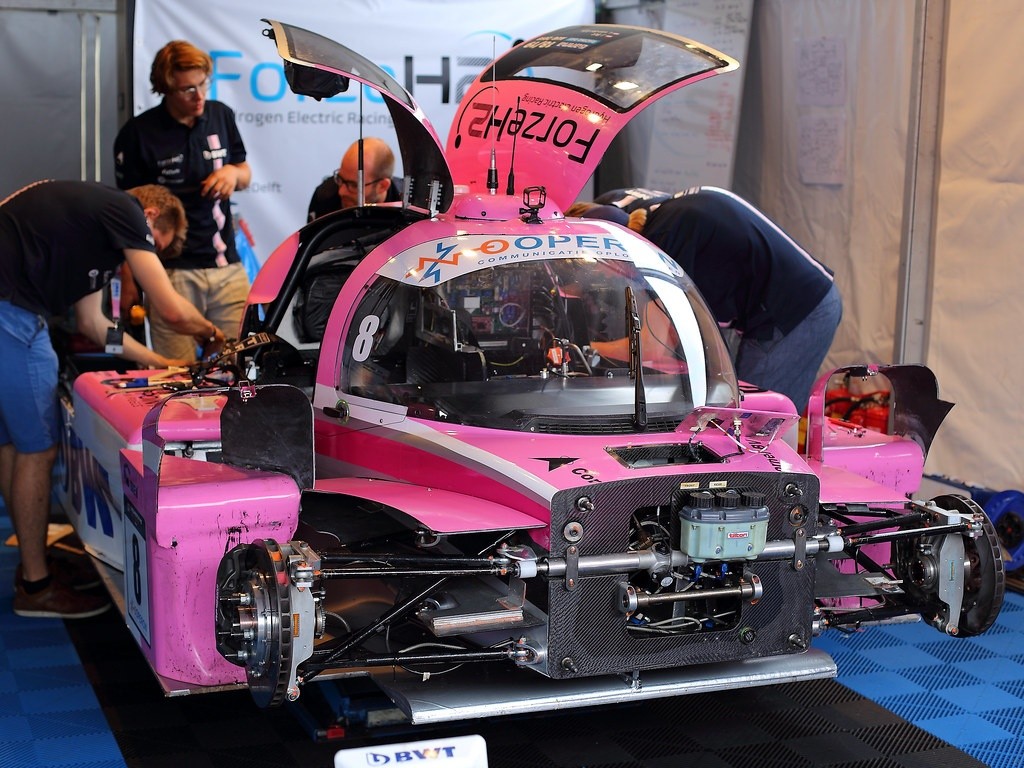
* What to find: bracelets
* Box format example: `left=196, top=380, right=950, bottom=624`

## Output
left=207, top=326, right=216, bottom=341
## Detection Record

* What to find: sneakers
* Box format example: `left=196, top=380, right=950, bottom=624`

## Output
left=12, top=560, right=111, bottom=619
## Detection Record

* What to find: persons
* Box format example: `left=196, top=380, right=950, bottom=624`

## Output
left=0, top=179, right=225, bottom=618
left=564, top=187, right=842, bottom=416
left=113, top=41, right=251, bottom=370
left=294, top=138, right=404, bottom=225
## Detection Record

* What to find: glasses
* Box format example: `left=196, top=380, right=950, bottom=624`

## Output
left=172, top=77, right=209, bottom=93
left=333, top=168, right=383, bottom=193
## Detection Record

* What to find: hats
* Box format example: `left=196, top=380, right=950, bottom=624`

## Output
left=580, top=205, right=630, bottom=227
